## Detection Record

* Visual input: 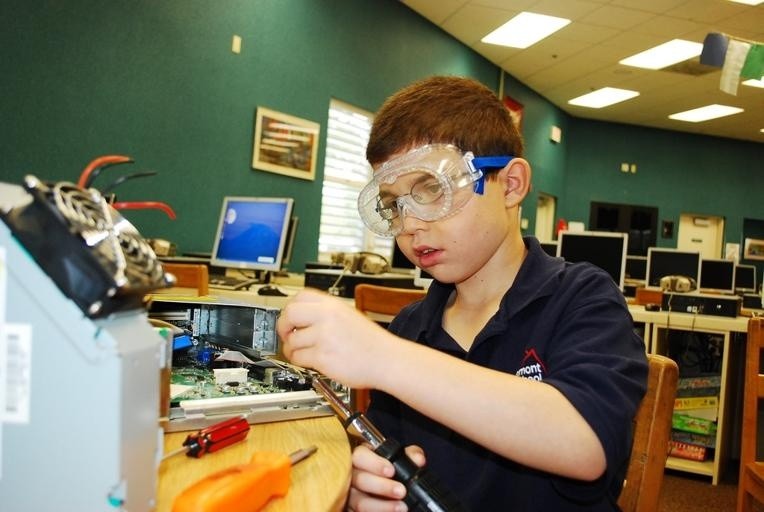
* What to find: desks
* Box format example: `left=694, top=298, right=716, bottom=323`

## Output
left=142, top=270, right=755, bottom=488
left=147, top=415, right=353, bottom=510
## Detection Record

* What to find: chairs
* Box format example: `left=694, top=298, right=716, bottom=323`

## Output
left=348, top=284, right=437, bottom=427
left=734, top=316, right=764, bottom=511
left=610, top=351, right=682, bottom=512
left=157, top=260, right=208, bottom=304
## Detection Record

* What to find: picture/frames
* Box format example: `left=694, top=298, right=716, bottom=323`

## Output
left=249, top=105, right=322, bottom=184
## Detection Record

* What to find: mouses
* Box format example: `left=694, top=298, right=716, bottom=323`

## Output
left=251, top=284, right=287, bottom=297
left=644, top=303, right=659, bottom=311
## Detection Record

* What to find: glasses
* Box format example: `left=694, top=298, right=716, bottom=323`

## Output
left=375, top=172, right=471, bottom=220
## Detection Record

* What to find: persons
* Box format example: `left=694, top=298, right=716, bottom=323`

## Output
left=277, top=76, right=650, bottom=512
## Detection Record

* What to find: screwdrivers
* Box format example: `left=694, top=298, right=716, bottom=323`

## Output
left=162, top=417, right=249, bottom=463
left=173, top=442, right=323, bottom=509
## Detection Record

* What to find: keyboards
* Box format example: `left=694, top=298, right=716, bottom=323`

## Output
left=211, top=276, right=260, bottom=292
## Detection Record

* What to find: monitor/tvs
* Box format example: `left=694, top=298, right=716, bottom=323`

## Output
left=624, top=255, right=649, bottom=283
left=645, top=247, right=702, bottom=296
left=209, top=196, right=295, bottom=272
left=274, top=216, right=299, bottom=279
left=735, top=264, right=757, bottom=293
left=699, top=259, right=735, bottom=294
left=539, top=240, right=558, bottom=257
left=555, top=231, right=629, bottom=294
